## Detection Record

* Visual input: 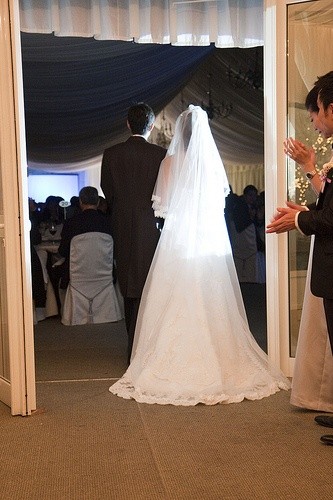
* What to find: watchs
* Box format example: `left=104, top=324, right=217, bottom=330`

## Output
left=305, top=169, right=317, bottom=181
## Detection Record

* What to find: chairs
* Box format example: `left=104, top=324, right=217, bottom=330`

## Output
left=229, top=220, right=266, bottom=283
left=62, top=232, right=122, bottom=325
left=32, top=250, right=58, bottom=324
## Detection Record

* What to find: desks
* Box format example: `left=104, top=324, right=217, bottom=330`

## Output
left=36, top=240, right=60, bottom=252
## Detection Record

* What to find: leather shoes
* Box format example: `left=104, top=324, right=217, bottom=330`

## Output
left=320, top=434, right=333, bottom=446
left=314, top=415, right=333, bottom=428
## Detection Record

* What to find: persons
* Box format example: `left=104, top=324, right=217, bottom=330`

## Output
left=29, top=185, right=106, bottom=309
left=100, top=101, right=169, bottom=368
left=281, top=83, right=333, bottom=412
left=265, top=72, right=333, bottom=445
left=109, top=103, right=292, bottom=407
left=224, top=185, right=265, bottom=254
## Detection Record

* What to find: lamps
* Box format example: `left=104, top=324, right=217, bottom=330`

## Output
left=156, top=107, right=174, bottom=148
left=198, top=59, right=233, bottom=121
